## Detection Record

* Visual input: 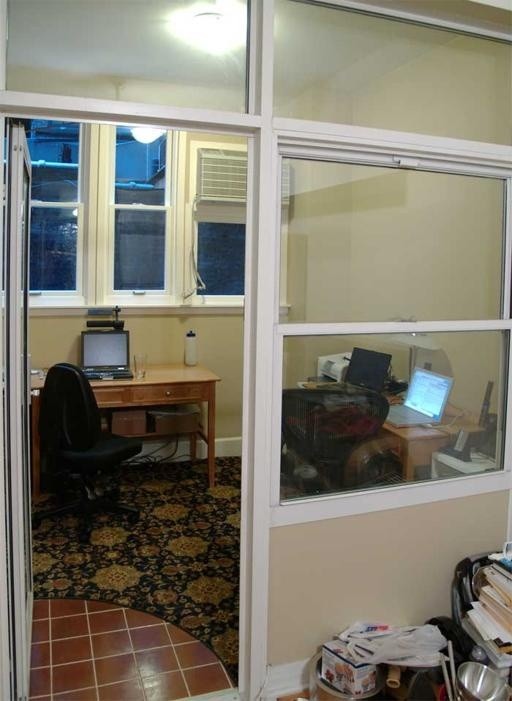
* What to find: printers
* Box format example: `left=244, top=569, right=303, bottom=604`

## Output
left=317, top=352, right=352, bottom=383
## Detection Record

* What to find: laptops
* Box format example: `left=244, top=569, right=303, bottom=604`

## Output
left=81, top=329, right=134, bottom=380
left=316, top=347, right=392, bottom=393
left=385, top=366, right=454, bottom=428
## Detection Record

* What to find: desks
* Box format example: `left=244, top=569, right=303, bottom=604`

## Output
left=302, top=382, right=486, bottom=482
left=28, top=362, right=222, bottom=505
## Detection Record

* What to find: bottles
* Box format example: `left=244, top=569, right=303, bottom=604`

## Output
left=185, top=331, right=197, bottom=366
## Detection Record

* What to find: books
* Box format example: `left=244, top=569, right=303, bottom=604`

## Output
left=463, top=551, right=511, bottom=691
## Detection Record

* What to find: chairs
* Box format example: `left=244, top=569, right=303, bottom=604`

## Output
left=283, top=389, right=390, bottom=496
left=31, top=361, right=143, bottom=544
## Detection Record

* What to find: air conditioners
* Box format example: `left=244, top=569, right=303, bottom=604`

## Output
left=196, top=149, right=292, bottom=206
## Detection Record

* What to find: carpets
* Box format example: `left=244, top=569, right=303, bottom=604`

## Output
left=30, top=455, right=330, bottom=687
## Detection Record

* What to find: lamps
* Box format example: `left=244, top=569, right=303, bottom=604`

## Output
left=129, top=126, right=166, bottom=144
left=388, top=315, right=440, bottom=382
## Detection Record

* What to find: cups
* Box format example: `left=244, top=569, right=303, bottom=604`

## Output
left=134, top=354, right=146, bottom=381
left=458, top=661, right=512, bottom=701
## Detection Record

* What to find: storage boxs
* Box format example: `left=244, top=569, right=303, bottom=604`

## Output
left=110, top=406, right=200, bottom=437
left=321, top=639, right=378, bottom=695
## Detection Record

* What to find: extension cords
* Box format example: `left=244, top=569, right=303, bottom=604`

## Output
left=140, top=462, right=162, bottom=472
